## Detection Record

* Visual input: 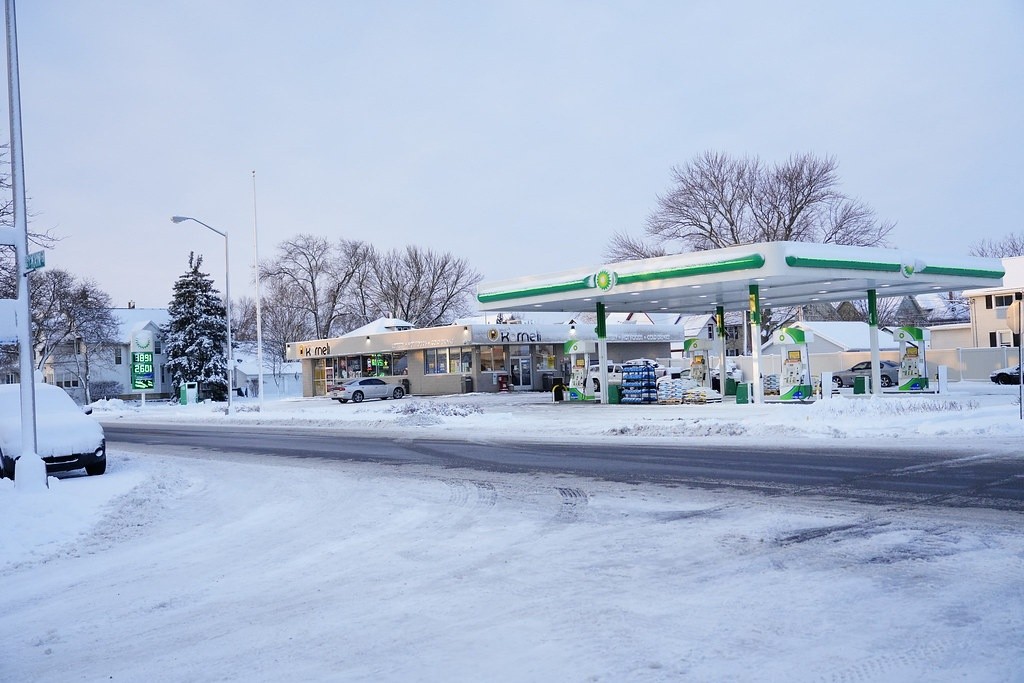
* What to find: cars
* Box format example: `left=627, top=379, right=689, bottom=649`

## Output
left=330, top=378, right=406, bottom=404
left=0, top=384, right=106, bottom=481
left=656, top=368, right=731, bottom=391
left=624, top=358, right=667, bottom=382
left=989, top=365, right=1024, bottom=384
left=832, top=360, right=900, bottom=388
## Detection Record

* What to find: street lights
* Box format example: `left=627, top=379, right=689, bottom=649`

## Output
left=171, top=216, right=236, bottom=416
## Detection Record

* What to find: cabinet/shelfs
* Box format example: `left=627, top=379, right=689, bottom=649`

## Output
left=620, top=367, right=658, bottom=403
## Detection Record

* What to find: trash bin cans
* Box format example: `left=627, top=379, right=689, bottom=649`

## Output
left=498, top=374, right=509, bottom=391
left=402, top=379, right=409, bottom=394
left=178, top=381, right=198, bottom=405
left=542, top=372, right=554, bottom=392
left=461, top=376, right=473, bottom=393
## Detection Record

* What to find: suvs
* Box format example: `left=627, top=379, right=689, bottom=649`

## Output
left=581, top=364, right=638, bottom=392
